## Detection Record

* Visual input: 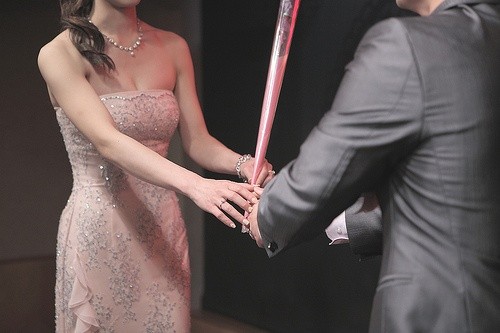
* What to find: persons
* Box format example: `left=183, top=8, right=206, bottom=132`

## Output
left=245, top=0, right=500, bottom=333
left=37, top=1, right=278, bottom=333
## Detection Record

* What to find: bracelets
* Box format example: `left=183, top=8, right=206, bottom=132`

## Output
left=235, top=153, right=252, bottom=179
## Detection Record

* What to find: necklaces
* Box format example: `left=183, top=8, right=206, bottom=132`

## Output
left=88, top=17, right=144, bottom=56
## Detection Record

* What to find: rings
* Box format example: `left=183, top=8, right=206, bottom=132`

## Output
left=268, top=170, right=276, bottom=175
